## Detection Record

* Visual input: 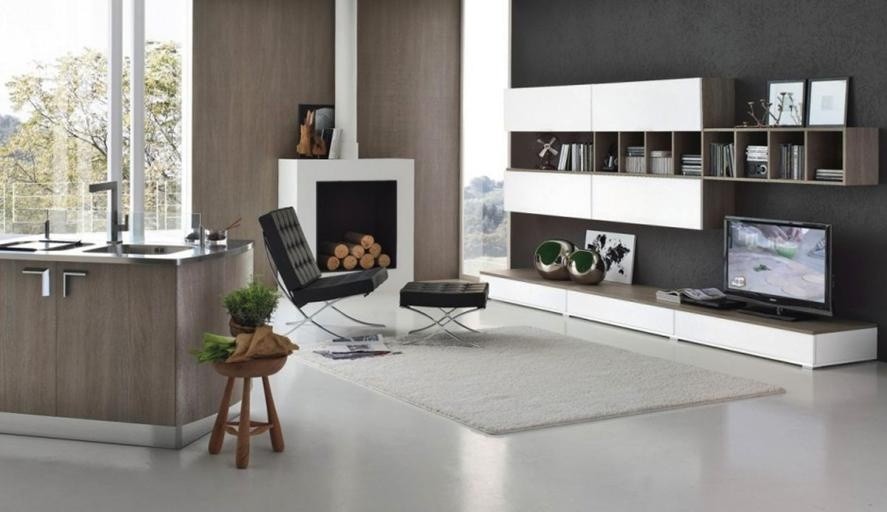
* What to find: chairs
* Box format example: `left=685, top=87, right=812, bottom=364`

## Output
left=258, top=205, right=388, bottom=341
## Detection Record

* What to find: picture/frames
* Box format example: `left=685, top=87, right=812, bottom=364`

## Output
left=767, top=80, right=807, bottom=127
left=296, top=103, right=336, bottom=159
left=807, top=77, right=850, bottom=128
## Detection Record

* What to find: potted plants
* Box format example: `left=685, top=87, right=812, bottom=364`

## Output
left=222, top=272, right=284, bottom=335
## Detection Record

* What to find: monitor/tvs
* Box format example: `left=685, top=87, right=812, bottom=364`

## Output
left=723, top=215, right=834, bottom=321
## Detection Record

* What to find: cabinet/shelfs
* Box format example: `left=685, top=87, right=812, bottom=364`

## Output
left=0, top=247, right=255, bottom=449
left=480, top=269, right=878, bottom=368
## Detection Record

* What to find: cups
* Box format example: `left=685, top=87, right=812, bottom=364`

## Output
left=184, top=212, right=202, bottom=245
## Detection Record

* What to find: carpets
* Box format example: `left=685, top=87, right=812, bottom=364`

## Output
left=289, top=325, right=787, bottom=435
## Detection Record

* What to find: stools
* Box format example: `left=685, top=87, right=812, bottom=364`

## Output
left=206, top=353, right=288, bottom=469
left=397, top=282, right=490, bottom=347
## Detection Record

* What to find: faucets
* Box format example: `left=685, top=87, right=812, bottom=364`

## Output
left=88, top=180, right=131, bottom=244
left=192, top=212, right=206, bottom=249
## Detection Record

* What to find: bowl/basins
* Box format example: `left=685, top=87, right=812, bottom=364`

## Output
left=206, top=243, right=226, bottom=253
left=203, top=227, right=228, bottom=242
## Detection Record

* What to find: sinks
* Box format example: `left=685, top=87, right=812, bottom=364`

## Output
left=80, top=240, right=197, bottom=256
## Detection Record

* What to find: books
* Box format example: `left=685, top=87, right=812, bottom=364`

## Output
left=555, top=141, right=843, bottom=183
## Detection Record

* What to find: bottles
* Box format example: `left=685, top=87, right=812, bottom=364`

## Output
left=778, top=142, right=804, bottom=177
